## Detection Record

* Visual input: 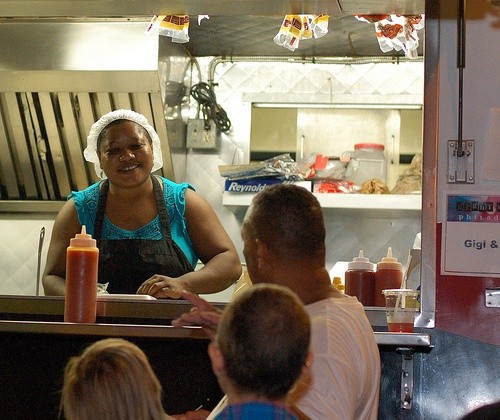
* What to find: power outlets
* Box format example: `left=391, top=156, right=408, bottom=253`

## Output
left=185, top=118, right=218, bottom=149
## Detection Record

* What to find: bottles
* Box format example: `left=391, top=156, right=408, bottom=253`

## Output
left=344, top=249, right=377, bottom=307
left=64, top=224, right=99, bottom=324
left=374, top=247, right=403, bottom=307
left=347, top=143, right=387, bottom=186
left=315, top=156, right=346, bottom=179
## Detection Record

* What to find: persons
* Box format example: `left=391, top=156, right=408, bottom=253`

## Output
left=42, top=109, right=243, bottom=300
left=62, top=339, right=171, bottom=420
left=171, top=184, right=381, bottom=420
left=210, top=284, right=312, bottom=420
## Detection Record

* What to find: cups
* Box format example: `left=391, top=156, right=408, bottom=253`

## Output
left=381, top=289, right=420, bottom=333
